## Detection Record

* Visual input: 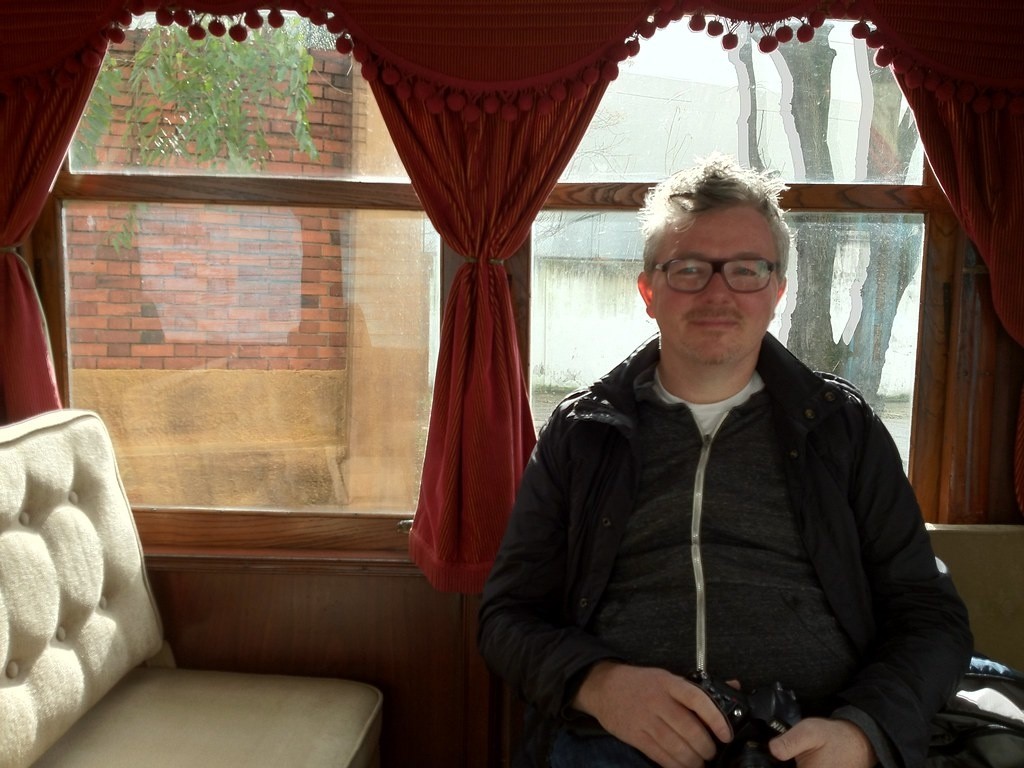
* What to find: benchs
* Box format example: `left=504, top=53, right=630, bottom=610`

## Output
left=0, top=408, right=387, bottom=767
left=919, top=523, right=1024, bottom=683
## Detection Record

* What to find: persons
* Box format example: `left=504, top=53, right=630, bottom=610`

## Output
left=474, top=163, right=974, bottom=768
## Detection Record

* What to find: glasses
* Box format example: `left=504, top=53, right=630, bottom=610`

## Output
left=656, top=255, right=781, bottom=294
